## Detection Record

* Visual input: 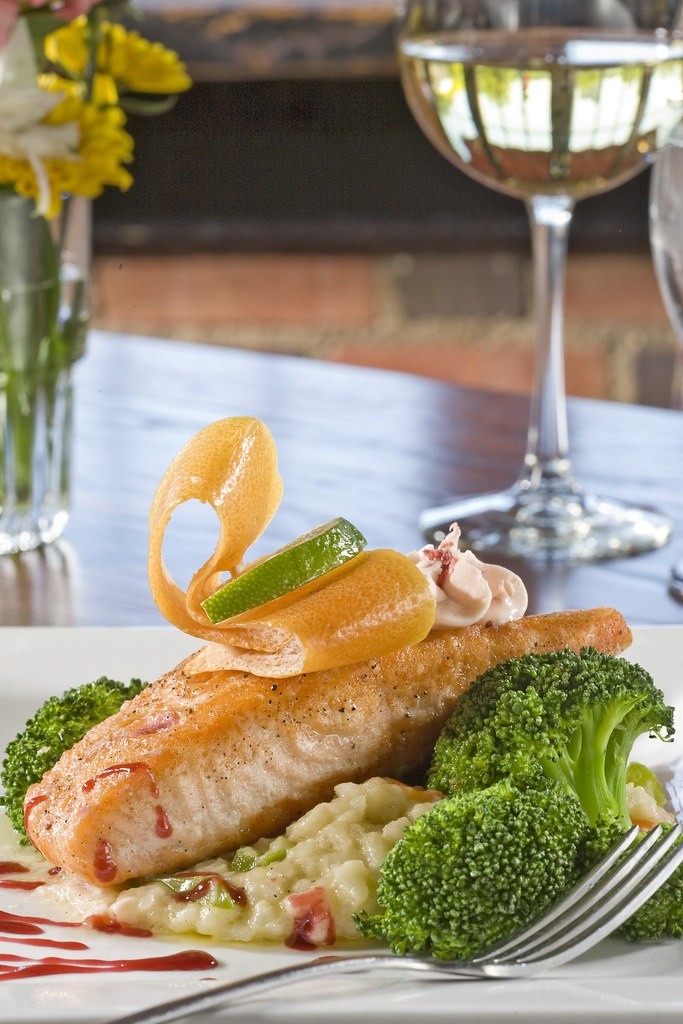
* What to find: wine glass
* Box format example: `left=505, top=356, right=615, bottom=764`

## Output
left=391, top=3, right=676, bottom=561
left=651, top=109, right=683, bottom=601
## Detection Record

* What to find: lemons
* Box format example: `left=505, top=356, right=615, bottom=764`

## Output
left=199, top=517, right=366, bottom=623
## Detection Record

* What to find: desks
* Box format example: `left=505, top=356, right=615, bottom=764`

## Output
left=0, top=328, right=683, bottom=629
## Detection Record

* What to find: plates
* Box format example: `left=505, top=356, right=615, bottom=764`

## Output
left=1, top=622, right=683, bottom=1024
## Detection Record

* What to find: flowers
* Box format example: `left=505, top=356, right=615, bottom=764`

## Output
left=0, top=0, right=194, bottom=225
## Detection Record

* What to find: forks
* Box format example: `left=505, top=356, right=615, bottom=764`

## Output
left=104, top=819, right=683, bottom=1024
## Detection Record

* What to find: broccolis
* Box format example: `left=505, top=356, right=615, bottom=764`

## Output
left=0, top=675, right=152, bottom=853
left=352, top=645, right=683, bottom=962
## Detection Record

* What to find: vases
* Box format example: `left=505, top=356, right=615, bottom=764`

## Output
left=0, top=196, right=84, bottom=555
